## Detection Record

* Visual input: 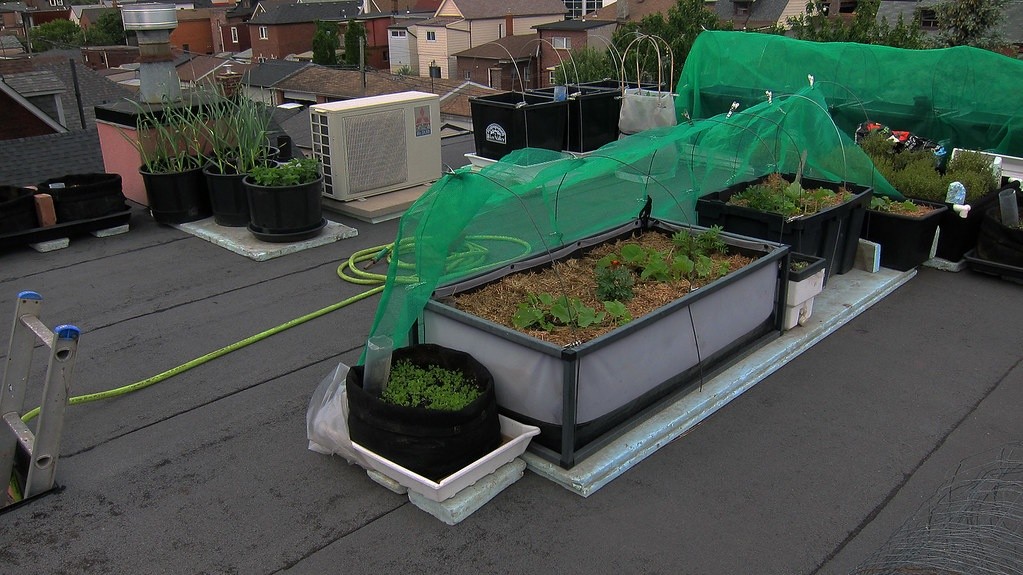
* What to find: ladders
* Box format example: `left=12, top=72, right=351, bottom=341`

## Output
left=0, top=292, right=79, bottom=509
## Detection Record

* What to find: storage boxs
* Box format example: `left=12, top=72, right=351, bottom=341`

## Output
left=464, top=31, right=667, bottom=161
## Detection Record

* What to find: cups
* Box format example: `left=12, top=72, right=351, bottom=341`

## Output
left=553, top=86, right=566, bottom=102
left=998, top=188, right=1019, bottom=227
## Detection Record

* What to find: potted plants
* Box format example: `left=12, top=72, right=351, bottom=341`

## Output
left=417, top=214, right=791, bottom=469
left=109, top=67, right=279, bottom=227
left=349, top=344, right=542, bottom=502
left=242, top=154, right=328, bottom=242
left=694, top=129, right=1023, bottom=326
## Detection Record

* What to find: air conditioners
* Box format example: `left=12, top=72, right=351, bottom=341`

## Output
left=311, top=90, right=444, bottom=202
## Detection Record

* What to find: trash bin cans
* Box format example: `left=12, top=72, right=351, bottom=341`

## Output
left=525, top=86, right=621, bottom=153
left=572, top=77, right=667, bottom=92
left=468, top=90, right=575, bottom=166
